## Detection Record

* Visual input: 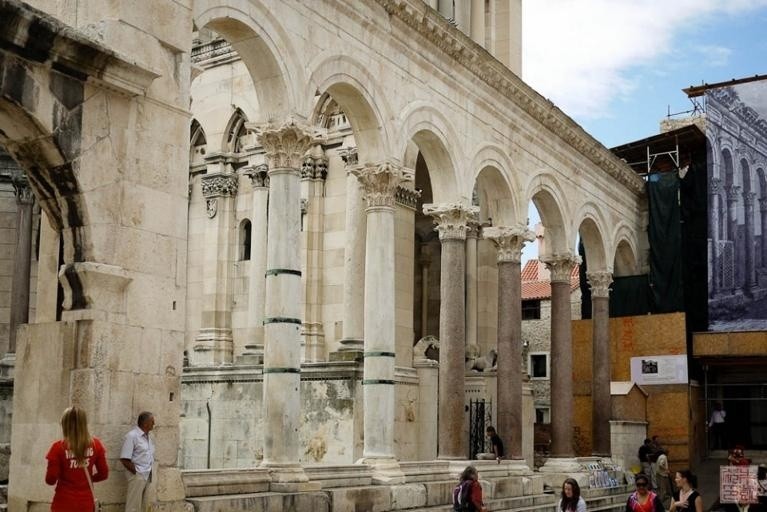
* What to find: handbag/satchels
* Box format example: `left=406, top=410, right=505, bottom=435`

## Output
left=93, top=499, right=102, bottom=512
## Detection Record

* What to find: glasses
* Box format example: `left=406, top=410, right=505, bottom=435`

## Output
left=635, top=482, right=647, bottom=488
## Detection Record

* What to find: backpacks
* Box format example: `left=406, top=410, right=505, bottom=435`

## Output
left=452, top=480, right=475, bottom=512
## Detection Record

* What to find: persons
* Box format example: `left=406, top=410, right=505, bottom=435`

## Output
left=624, top=435, right=766, bottom=512
left=557, top=477, right=586, bottom=512
left=495, top=456, right=498, bottom=459
left=475, top=426, right=504, bottom=464
left=707, top=402, right=728, bottom=451
left=120, top=411, right=157, bottom=512
left=43, top=404, right=109, bottom=512
left=452, top=465, right=486, bottom=512
